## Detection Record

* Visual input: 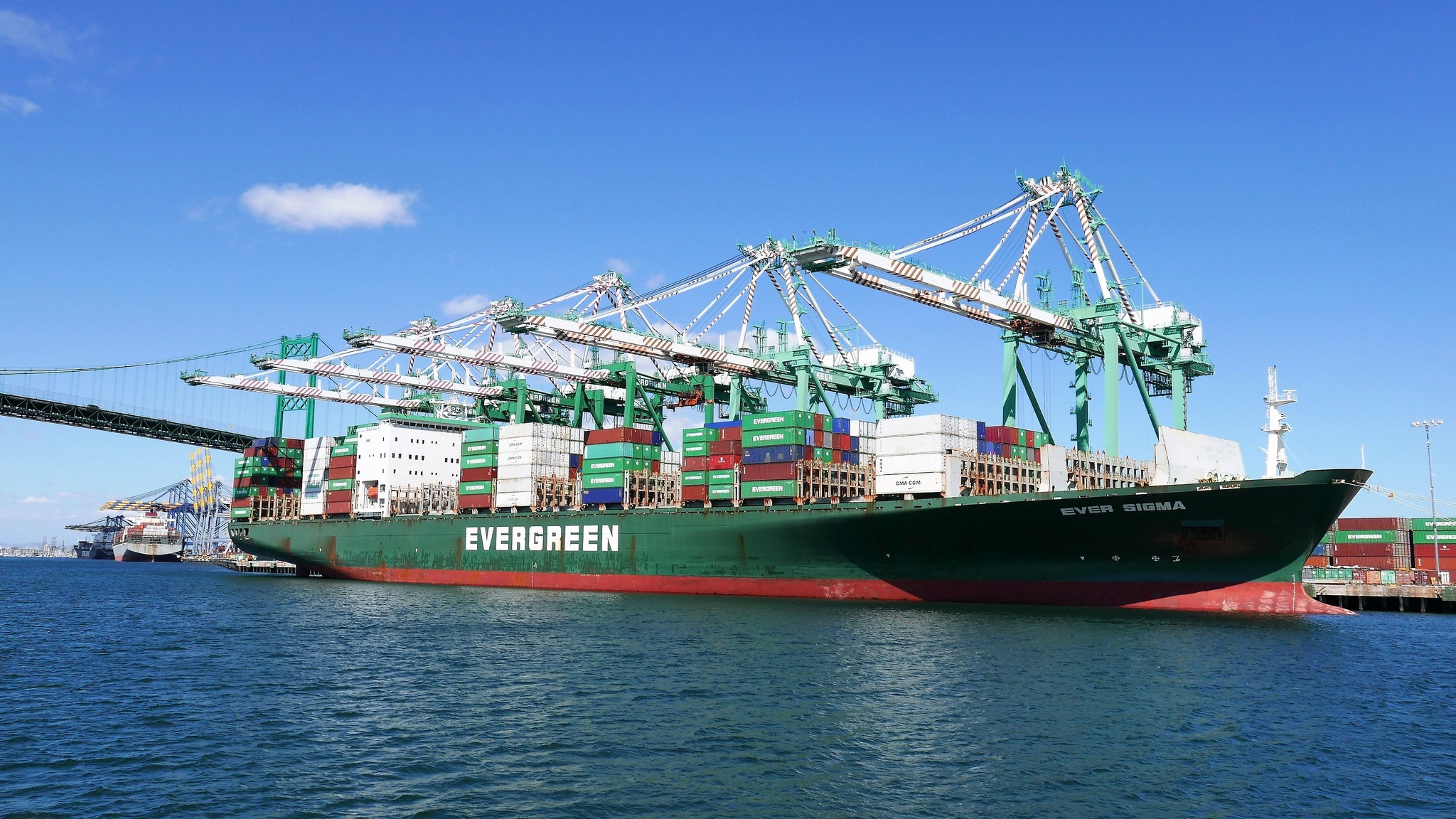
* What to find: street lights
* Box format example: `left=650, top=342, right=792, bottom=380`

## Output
left=1410, top=420, right=1444, bottom=571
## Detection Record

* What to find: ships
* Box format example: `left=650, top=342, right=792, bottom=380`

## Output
left=70, top=516, right=133, bottom=560
left=174, top=163, right=1384, bottom=616
left=112, top=509, right=183, bottom=561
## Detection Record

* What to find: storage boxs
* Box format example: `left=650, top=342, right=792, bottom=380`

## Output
left=185, top=545, right=256, bottom=561
left=226, top=412, right=1047, bottom=522
left=114, top=513, right=182, bottom=544
left=1302, top=516, right=1456, bottom=586
